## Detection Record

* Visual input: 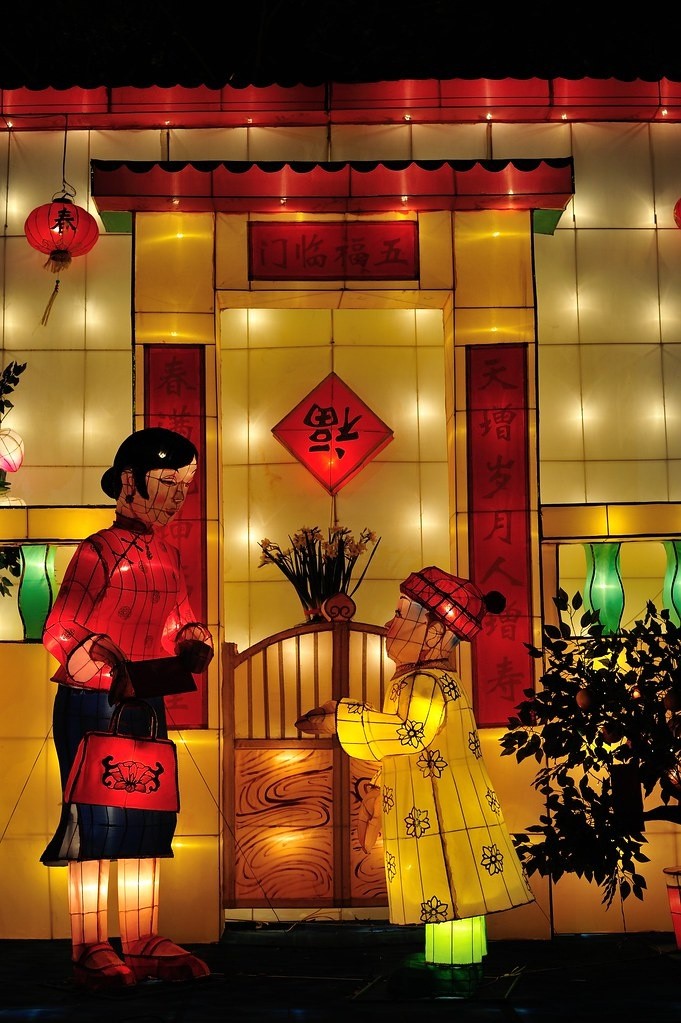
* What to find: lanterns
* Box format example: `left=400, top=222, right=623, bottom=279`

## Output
left=293, top=566, right=536, bottom=971
left=38, top=427, right=216, bottom=984
left=24, top=198, right=99, bottom=328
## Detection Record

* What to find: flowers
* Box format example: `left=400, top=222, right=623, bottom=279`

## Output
left=255, top=524, right=382, bottom=625
